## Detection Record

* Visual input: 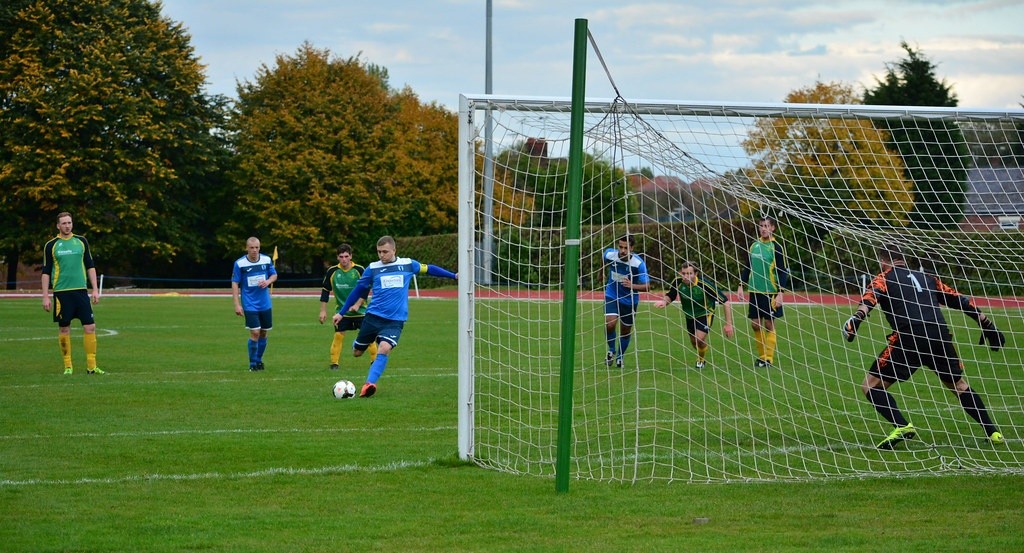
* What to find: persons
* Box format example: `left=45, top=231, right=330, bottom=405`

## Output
left=841, top=244, right=1007, bottom=450
left=653, top=261, right=733, bottom=370
left=333, top=235, right=458, bottom=397
left=319, top=244, right=378, bottom=370
left=40, top=212, right=104, bottom=375
left=737, top=213, right=789, bottom=367
left=599, top=234, right=649, bottom=367
left=232, top=237, right=278, bottom=373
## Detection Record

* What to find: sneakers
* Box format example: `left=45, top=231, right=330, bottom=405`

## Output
left=615, top=353, right=624, bottom=367
left=359, top=383, right=377, bottom=398
left=250, top=365, right=257, bottom=372
left=64, top=367, right=74, bottom=375
left=983, top=432, right=1004, bottom=444
left=256, top=362, right=264, bottom=370
left=695, top=358, right=706, bottom=368
left=755, top=358, right=772, bottom=368
left=604, top=350, right=616, bottom=366
left=86, top=366, right=105, bottom=375
left=875, top=422, right=917, bottom=451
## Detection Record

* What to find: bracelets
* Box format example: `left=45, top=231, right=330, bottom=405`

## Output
left=738, top=283, right=744, bottom=287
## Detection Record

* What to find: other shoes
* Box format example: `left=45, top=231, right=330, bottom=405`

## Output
left=329, top=364, right=338, bottom=370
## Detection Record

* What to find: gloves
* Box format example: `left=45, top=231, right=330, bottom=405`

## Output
left=978, top=316, right=1006, bottom=352
left=841, top=310, right=866, bottom=342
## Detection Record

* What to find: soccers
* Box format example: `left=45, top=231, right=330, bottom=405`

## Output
left=332, top=380, right=356, bottom=399
